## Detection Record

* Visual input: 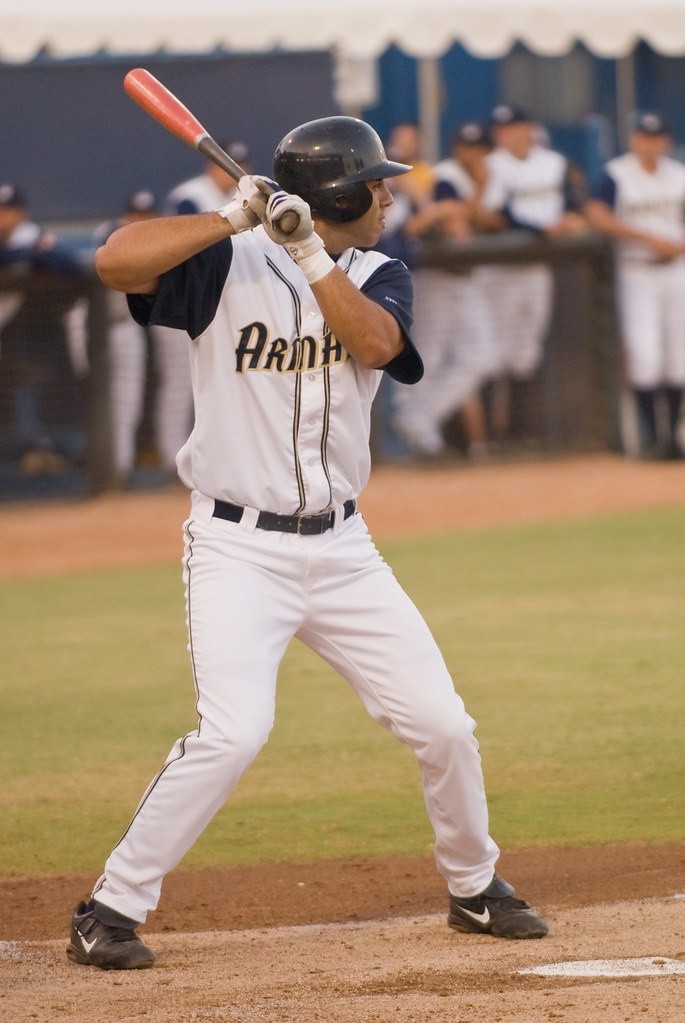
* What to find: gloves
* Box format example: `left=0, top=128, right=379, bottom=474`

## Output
left=249, top=189, right=336, bottom=285
left=215, top=175, right=281, bottom=235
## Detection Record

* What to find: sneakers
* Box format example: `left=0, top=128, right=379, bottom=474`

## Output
left=446, top=877, right=549, bottom=939
left=65, top=901, right=155, bottom=971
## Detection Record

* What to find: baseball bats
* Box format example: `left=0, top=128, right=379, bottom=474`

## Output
left=123, top=67, right=300, bottom=233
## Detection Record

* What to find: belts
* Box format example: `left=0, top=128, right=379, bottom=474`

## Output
left=211, top=491, right=357, bottom=537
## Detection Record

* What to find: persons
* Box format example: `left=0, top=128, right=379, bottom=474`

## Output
left=66, top=116, right=548, bottom=970
left=0, top=104, right=685, bottom=500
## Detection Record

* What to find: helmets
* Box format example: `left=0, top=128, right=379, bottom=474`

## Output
left=273, top=115, right=414, bottom=224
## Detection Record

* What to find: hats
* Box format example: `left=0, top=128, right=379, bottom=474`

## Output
left=122, top=191, right=158, bottom=214
left=440, top=103, right=673, bottom=148
left=0, top=185, right=27, bottom=210
left=223, top=141, right=251, bottom=166
